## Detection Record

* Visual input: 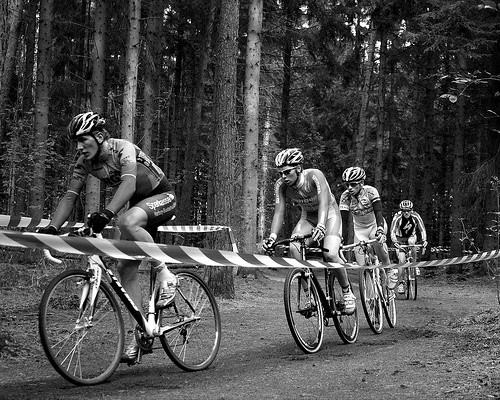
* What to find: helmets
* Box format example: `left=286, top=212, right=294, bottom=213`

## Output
left=399, top=200, right=413, bottom=210
left=341, top=167, right=366, bottom=185
left=66, top=111, right=106, bottom=143
left=275, top=148, right=304, bottom=168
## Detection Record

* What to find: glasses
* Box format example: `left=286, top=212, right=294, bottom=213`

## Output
left=345, top=181, right=361, bottom=188
left=278, top=166, right=297, bottom=177
left=402, top=209, right=411, bottom=212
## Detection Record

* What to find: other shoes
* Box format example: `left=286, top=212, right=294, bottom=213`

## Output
left=342, top=288, right=356, bottom=314
left=387, top=269, right=399, bottom=289
left=416, top=267, right=421, bottom=276
left=398, top=284, right=404, bottom=293
left=303, top=292, right=314, bottom=309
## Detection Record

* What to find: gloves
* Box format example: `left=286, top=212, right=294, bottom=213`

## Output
left=87, top=207, right=115, bottom=234
left=262, top=233, right=277, bottom=251
left=311, top=223, right=327, bottom=242
left=374, top=228, right=387, bottom=244
left=38, top=225, right=59, bottom=235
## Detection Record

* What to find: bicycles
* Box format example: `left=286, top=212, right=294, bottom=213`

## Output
left=399, top=241, right=424, bottom=301
left=39, top=214, right=221, bottom=385
left=338, top=233, right=397, bottom=335
left=265, top=228, right=359, bottom=354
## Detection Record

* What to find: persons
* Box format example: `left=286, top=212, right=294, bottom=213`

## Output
left=263, top=149, right=357, bottom=315
left=35, top=112, right=179, bottom=363
left=391, top=200, right=427, bottom=293
left=339, top=167, right=400, bottom=309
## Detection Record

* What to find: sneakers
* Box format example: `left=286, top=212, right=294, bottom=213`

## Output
left=155, top=275, right=180, bottom=307
left=121, top=334, right=138, bottom=359
